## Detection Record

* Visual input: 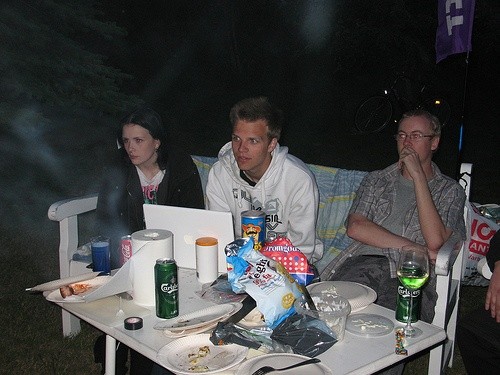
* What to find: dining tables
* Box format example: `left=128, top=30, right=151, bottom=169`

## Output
left=43, top=268, right=446, bottom=375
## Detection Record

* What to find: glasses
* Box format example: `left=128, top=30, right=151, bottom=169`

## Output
left=393, top=132, right=435, bottom=141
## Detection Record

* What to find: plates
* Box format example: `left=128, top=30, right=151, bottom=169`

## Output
left=234, top=353, right=334, bottom=375
left=158, top=333, right=249, bottom=375
left=153, top=303, right=235, bottom=330
left=46, top=279, right=109, bottom=302
left=163, top=302, right=243, bottom=338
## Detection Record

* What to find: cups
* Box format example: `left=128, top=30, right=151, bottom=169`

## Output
left=90, top=235, right=112, bottom=276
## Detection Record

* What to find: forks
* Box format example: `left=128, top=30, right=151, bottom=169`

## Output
left=252, top=357, right=322, bottom=375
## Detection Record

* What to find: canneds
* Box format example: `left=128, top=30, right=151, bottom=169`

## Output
left=395, top=261, right=420, bottom=323
left=119, top=235, right=132, bottom=266
left=154, top=258, right=179, bottom=320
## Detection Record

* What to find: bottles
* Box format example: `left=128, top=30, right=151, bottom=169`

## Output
left=196, top=237, right=217, bottom=283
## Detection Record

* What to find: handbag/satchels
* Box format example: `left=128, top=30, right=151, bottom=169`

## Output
left=460, top=200, right=500, bottom=287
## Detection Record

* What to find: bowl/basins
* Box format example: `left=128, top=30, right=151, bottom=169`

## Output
left=295, top=293, right=351, bottom=343
left=304, top=282, right=376, bottom=316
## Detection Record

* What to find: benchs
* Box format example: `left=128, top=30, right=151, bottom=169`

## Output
left=48, top=163, right=473, bottom=375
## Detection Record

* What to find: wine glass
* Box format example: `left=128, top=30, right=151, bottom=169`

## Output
left=394, top=245, right=429, bottom=338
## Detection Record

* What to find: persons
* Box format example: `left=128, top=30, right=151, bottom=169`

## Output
left=455, top=229, right=500, bottom=375
left=204, top=97, right=324, bottom=284
left=326, top=109, right=467, bottom=375
left=94, top=106, right=205, bottom=375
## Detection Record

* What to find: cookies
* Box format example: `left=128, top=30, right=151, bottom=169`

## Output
left=277, top=264, right=303, bottom=309
